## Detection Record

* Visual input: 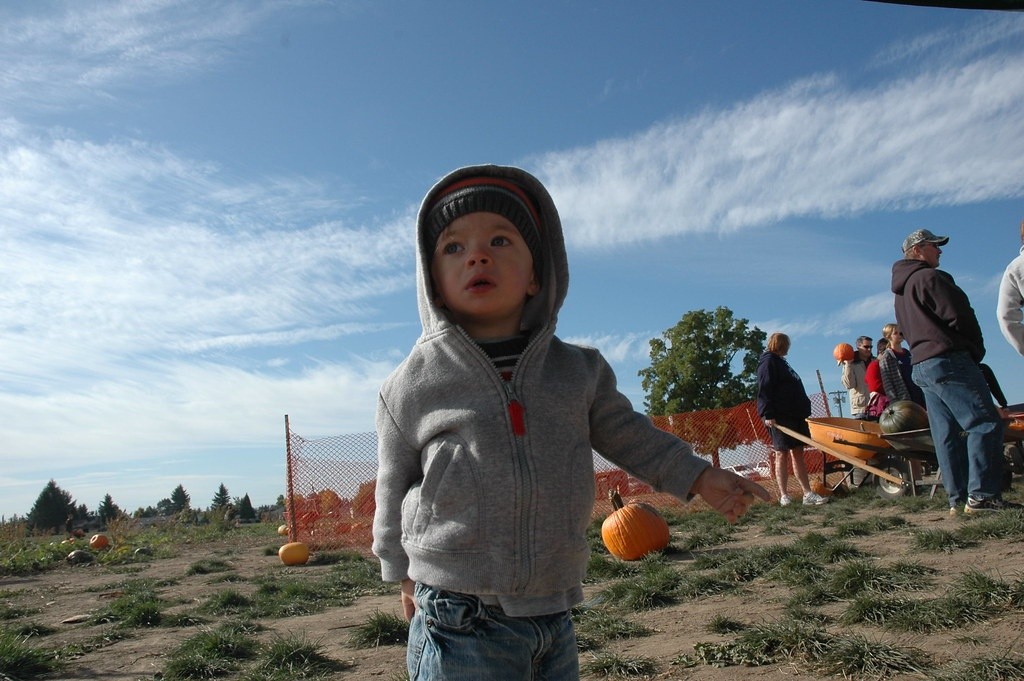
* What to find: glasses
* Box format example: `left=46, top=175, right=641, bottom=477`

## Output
left=863, top=345, right=873, bottom=348
left=918, top=243, right=939, bottom=249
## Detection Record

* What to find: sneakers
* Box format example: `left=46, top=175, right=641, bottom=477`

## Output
left=802, top=492, right=829, bottom=505
left=780, top=493, right=792, bottom=507
left=950, top=505, right=963, bottom=516
left=964, top=494, right=1024, bottom=515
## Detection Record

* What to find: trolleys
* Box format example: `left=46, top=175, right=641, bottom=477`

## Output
left=803, top=413, right=1024, bottom=501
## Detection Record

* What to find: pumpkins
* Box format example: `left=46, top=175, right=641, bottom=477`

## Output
left=65, top=550, right=93, bottom=564
left=135, top=548, right=152, bottom=557
left=833, top=343, right=854, bottom=366
left=89, top=534, right=109, bottom=548
left=278, top=525, right=288, bottom=534
left=1009, top=421, right=1024, bottom=431
left=810, top=476, right=832, bottom=495
left=602, top=489, right=670, bottom=561
left=879, top=400, right=929, bottom=433
left=279, top=542, right=309, bottom=565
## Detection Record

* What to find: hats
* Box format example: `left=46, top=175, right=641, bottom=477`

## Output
left=902, top=228, right=949, bottom=253
left=424, top=175, right=544, bottom=305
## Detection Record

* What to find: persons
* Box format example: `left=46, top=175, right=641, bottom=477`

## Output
left=369, top=163, right=770, bottom=681
left=758, top=333, right=830, bottom=505
left=840, top=322, right=1010, bottom=423
left=892, top=228, right=1024, bottom=515
left=996, top=217, right=1024, bottom=356
